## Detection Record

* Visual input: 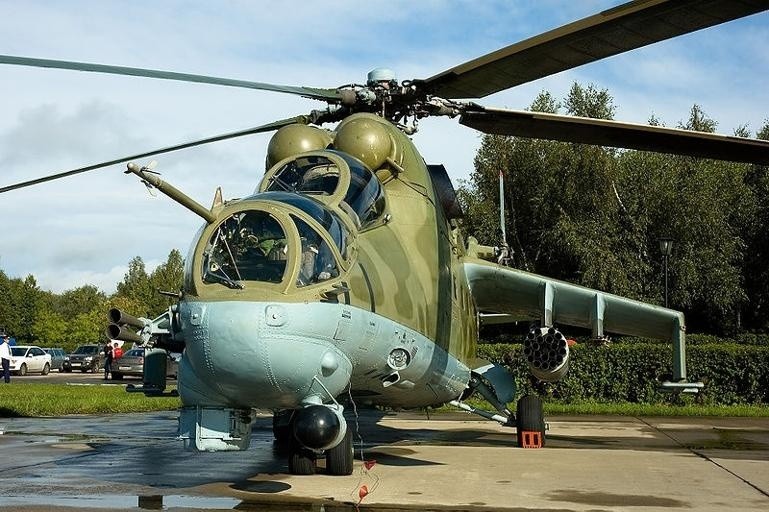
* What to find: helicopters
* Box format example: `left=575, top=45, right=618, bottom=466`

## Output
left=0, top=2, right=769, bottom=477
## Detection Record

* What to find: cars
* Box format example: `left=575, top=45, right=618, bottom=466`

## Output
left=0, top=344, right=179, bottom=380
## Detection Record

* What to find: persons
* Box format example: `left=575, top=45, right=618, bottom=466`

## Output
left=103, top=340, right=123, bottom=380
left=0, top=335, right=15, bottom=383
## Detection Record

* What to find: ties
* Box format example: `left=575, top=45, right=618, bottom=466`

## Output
left=8, top=345, right=11, bottom=355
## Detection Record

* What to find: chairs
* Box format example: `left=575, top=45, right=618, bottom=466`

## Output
left=266, top=238, right=318, bottom=286
